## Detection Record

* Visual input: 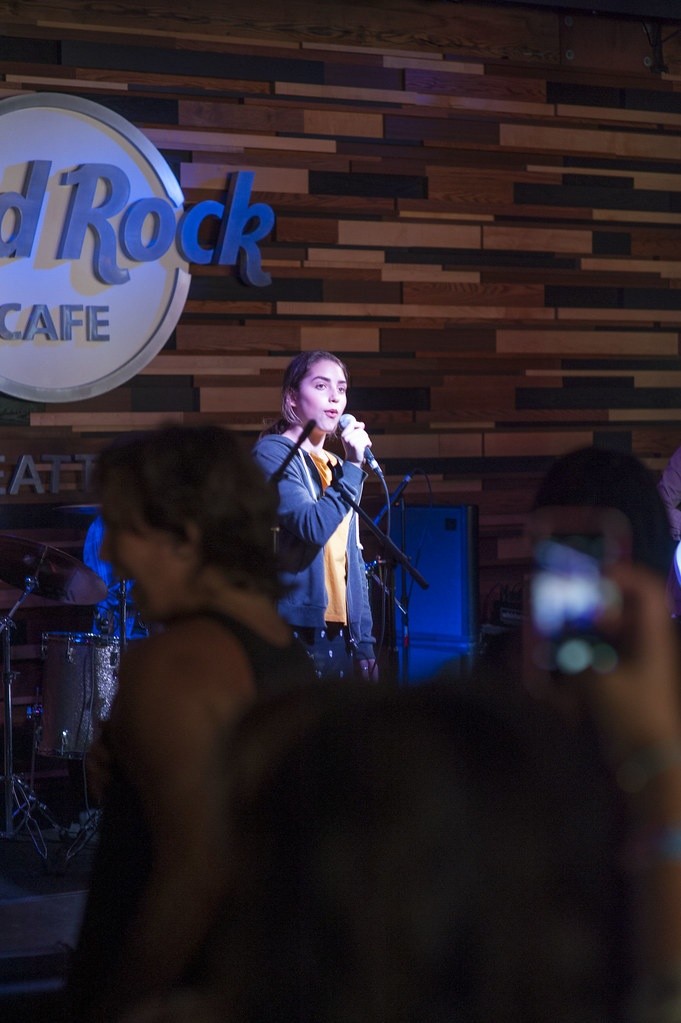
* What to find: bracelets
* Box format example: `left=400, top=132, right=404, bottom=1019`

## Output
left=617, top=737, right=679, bottom=787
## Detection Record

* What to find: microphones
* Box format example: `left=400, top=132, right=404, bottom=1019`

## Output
left=338, top=414, right=384, bottom=480
left=375, top=472, right=414, bottom=528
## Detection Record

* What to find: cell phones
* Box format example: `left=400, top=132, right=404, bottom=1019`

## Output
left=541, top=532, right=608, bottom=687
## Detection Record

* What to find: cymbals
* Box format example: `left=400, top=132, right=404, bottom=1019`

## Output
left=0, top=533, right=108, bottom=605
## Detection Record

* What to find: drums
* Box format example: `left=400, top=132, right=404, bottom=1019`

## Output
left=34, top=628, right=146, bottom=762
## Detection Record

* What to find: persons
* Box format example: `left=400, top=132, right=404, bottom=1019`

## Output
left=248, top=351, right=379, bottom=685
left=529, top=441, right=677, bottom=572
left=654, top=443, right=681, bottom=542
left=215, top=561, right=680, bottom=1023
left=73, top=420, right=308, bottom=1023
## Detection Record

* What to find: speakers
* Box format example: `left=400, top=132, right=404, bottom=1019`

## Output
left=386, top=503, right=482, bottom=642
left=475, top=614, right=528, bottom=689
left=388, top=637, right=480, bottom=691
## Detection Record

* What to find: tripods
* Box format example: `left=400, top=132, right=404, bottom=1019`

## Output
left=0, top=558, right=78, bottom=866
